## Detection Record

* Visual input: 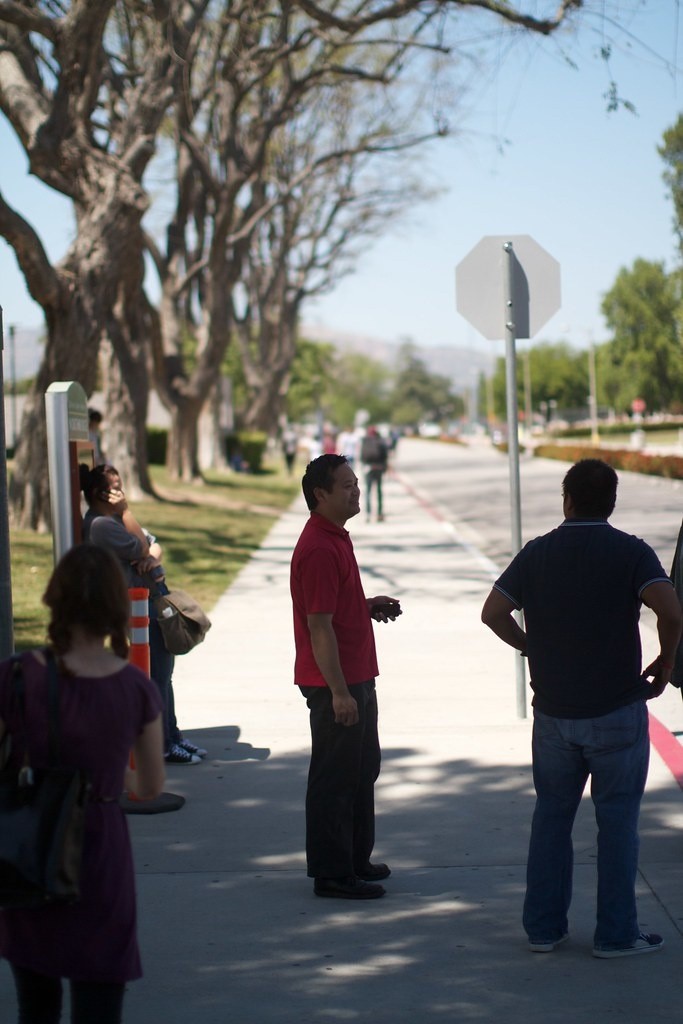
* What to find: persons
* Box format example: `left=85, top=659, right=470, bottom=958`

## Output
left=480, top=457, right=683, bottom=960
left=0, top=539, right=167, bottom=1024
left=276, top=408, right=397, bottom=474
left=289, top=452, right=404, bottom=901
left=88, top=409, right=111, bottom=467
left=359, top=425, right=390, bottom=523
left=77, top=463, right=208, bottom=766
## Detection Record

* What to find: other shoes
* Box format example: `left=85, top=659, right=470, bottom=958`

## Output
left=353, top=863, right=392, bottom=882
left=314, top=875, right=387, bottom=900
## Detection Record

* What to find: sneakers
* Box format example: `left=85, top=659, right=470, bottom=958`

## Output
left=528, top=925, right=571, bottom=953
left=592, top=929, right=666, bottom=959
left=180, top=740, right=208, bottom=758
left=163, top=743, right=204, bottom=766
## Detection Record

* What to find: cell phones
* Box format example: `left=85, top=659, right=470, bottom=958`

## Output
left=94, top=488, right=111, bottom=502
left=371, top=604, right=400, bottom=619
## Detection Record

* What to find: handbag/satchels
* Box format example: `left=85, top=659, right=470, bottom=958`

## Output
left=156, top=590, right=212, bottom=657
left=0, top=643, right=101, bottom=929
